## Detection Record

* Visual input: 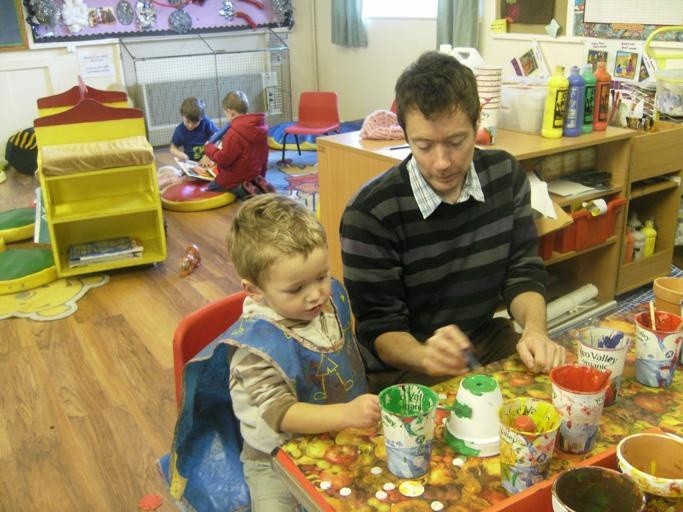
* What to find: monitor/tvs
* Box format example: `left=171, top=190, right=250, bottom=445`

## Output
left=69, top=236, right=144, bottom=268
left=175, top=156, right=218, bottom=180
left=34, top=187, right=50, bottom=244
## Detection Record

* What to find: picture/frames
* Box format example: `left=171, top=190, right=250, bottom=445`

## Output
left=266, top=302, right=683, bottom=512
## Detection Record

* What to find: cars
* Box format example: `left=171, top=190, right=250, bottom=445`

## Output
left=442, top=373, right=507, bottom=459
left=498, top=396, right=561, bottom=495
left=551, top=466, right=647, bottom=512
left=633, top=309, right=681, bottom=389
left=474, top=65, right=504, bottom=148
left=652, top=277, right=683, bottom=314
left=378, top=383, right=437, bottom=480
left=614, top=432, right=683, bottom=511
left=575, top=325, right=631, bottom=409
left=548, top=359, right=609, bottom=459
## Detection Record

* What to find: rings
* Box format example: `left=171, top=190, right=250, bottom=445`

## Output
left=391, top=145, right=410, bottom=149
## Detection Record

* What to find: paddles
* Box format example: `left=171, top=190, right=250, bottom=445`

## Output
left=233, top=148, right=320, bottom=221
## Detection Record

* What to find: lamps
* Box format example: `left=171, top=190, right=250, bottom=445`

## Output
left=280, top=90, right=341, bottom=165
left=172, top=289, right=249, bottom=511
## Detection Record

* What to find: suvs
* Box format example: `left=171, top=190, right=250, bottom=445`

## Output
left=549, top=363, right=611, bottom=455
left=551, top=465, right=647, bottom=512
left=576, top=325, right=629, bottom=407
left=652, top=67, right=683, bottom=117
left=652, top=277, right=683, bottom=369
left=443, top=373, right=507, bottom=457
left=634, top=310, right=683, bottom=389
left=498, top=397, right=562, bottom=497
left=438, top=44, right=486, bottom=72
left=377, top=383, right=439, bottom=479
left=615, top=433, right=683, bottom=512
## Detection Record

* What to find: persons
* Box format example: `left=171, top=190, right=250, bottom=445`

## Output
left=226, top=192, right=381, bottom=512
left=206, top=92, right=276, bottom=201
left=169, top=96, right=219, bottom=175
left=339, top=50, right=567, bottom=389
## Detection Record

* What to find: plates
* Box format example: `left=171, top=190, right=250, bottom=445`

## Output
left=612, top=119, right=682, bottom=298
left=31, top=95, right=166, bottom=278
left=313, top=116, right=636, bottom=332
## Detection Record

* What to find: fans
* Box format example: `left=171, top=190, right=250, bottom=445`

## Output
left=0, top=0, right=29, bottom=52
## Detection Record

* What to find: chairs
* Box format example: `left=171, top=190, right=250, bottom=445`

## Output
left=252, top=174, right=277, bottom=194
left=237, top=180, right=264, bottom=199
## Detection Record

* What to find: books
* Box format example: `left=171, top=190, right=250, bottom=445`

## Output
left=499, top=81, right=547, bottom=136
left=554, top=194, right=627, bottom=255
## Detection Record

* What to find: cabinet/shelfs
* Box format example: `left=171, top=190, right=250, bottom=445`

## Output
left=540, top=61, right=613, bottom=138
left=625, top=210, right=658, bottom=263
